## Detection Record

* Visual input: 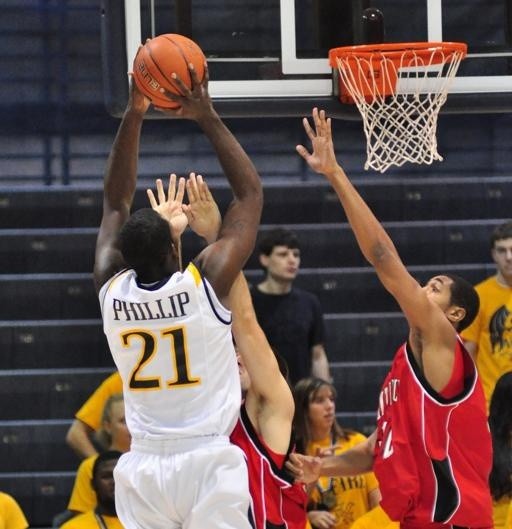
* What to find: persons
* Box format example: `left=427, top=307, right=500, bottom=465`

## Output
left=290, top=372, right=385, bottom=528
left=66, top=367, right=124, bottom=461
left=457, top=223, right=511, bottom=421
left=486, top=368, right=512, bottom=529
left=89, top=35, right=265, bottom=529
left=53, top=449, right=126, bottom=529
left=246, top=224, right=334, bottom=455
left=143, top=168, right=306, bottom=529
left=283, top=105, right=498, bottom=529
left=65, top=395, right=132, bottom=515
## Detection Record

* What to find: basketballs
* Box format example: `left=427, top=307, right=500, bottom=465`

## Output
left=134, top=33, right=207, bottom=110
